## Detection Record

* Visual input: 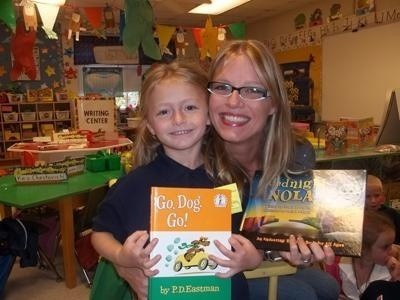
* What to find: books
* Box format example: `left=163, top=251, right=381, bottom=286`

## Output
left=147, top=183, right=235, bottom=300
left=237, top=164, right=368, bottom=258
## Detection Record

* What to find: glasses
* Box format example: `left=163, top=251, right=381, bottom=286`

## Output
left=206, top=80, right=271, bottom=101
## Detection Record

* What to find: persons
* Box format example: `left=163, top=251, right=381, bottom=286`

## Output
left=324, top=208, right=400, bottom=300
left=365, top=173, right=400, bottom=245
left=110, top=36, right=344, bottom=299
left=90, top=56, right=265, bottom=298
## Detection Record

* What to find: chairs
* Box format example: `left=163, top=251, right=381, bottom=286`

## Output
left=19, top=183, right=109, bottom=288
left=109, top=179, right=297, bottom=300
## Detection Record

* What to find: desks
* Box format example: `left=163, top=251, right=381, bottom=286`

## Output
left=0, top=167, right=124, bottom=289
left=315, top=146, right=400, bottom=169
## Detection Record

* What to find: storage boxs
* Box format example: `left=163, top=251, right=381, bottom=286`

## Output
left=3, top=91, right=70, bottom=141
left=126, top=118, right=142, bottom=128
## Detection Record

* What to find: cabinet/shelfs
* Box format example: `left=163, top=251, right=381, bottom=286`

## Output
left=0, top=101, right=73, bottom=160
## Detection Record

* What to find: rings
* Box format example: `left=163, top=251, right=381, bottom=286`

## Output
left=303, top=260, right=310, bottom=263
